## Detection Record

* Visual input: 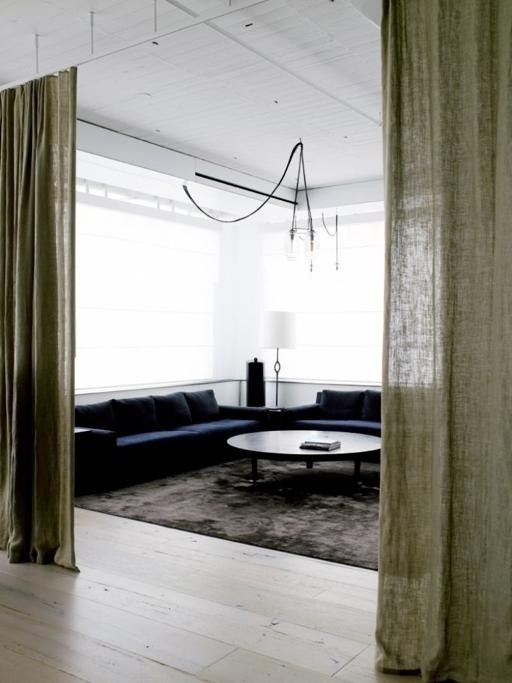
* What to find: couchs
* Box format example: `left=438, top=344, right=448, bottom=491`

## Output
left=76, top=387, right=273, bottom=496
left=280, top=391, right=381, bottom=462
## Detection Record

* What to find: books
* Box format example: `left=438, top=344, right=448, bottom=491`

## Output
left=300, top=438, right=340, bottom=450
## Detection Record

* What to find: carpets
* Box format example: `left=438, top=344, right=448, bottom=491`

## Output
left=74, top=455, right=381, bottom=570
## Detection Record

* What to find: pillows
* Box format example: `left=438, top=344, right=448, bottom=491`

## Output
left=319, top=389, right=381, bottom=422
left=75, top=389, right=221, bottom=435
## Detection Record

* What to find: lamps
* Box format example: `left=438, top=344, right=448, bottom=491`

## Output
left=256, top=308, right=297, bottom=409
